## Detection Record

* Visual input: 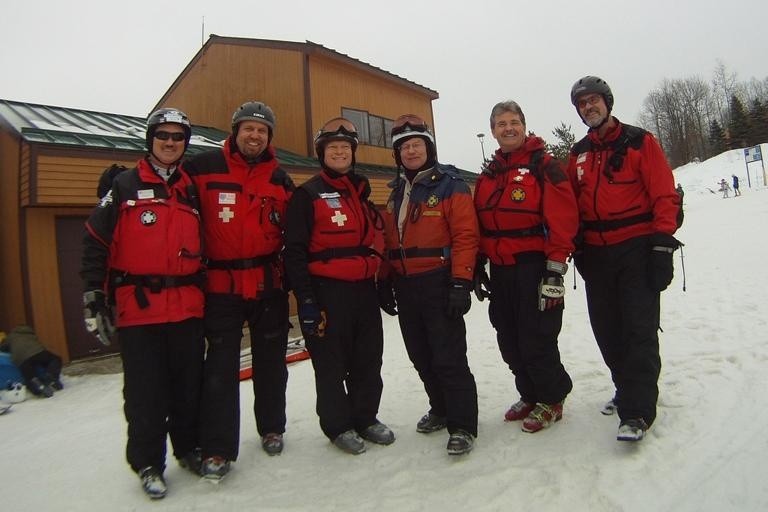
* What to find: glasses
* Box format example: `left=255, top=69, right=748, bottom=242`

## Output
left=314, top=116, right=359, bottom=143
left=572, top=95, right=604, bottom=110
left=148, top=130, right=185, bottom=142
left=391, top=114, right=435, bottom=138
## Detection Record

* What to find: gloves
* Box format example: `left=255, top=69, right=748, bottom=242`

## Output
left=472, top=252, right=495, bottom=302
left=263, top=289, right=293, bottom=335
left=639, top=230, right=684, bottom=296
left=91, top=161, right=129, bottom=199
left=441, top=278, right=473, bottom=321
left=79, top=288, right=117, bottom=348
left=291, top=289, right=329, bottom=341
left=535, top=259, right=570, bottom=314
left=375, top=277, right=400, bottom=317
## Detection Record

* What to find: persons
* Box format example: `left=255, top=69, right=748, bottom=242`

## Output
left=82, top=105, right=208, bottom=500
left=717, top=174, right=741, bottom=198
left=471, top=99, right=580, bottom=434
left=0, top=324, right=64, bottom=398
left=95, top=100, right=298, bottom=480
left=675, top=182, right=684, bottom=197
left=567, top=74, right=680, bottom=441
left=376, top=113, right=480, bottom=457
left=282, top=117, right=394, bottom=455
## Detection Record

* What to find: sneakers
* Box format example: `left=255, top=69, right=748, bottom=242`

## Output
left=329, top=426, right=368, bottom=455
left=175, top=442, right=235, bottom=483
left=444, top=428, right=476, bottom=456
left=30, top=374, right=65, bottom=398
left=504, top=397, right=537, bottom=421
left=520, top=399, right=566, bottom=433
left=615, top=412, right=652, bottom=445
left=136, top=462, right=170, bottom=501
left=599, top=393, right=621, bottom=417
left=357, top=417, right=396, bottom=446
left=414, top=408, right=447, bottom=434
left=260, top=428, right=286, bottom=456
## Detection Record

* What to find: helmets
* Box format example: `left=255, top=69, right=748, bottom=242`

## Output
left=390, top=113, right=436, bottom=148
left=570, top=73, right=615, bottom=112
left=230, top=100, right=276, bottom=133
left=144, top=106, right=193, bottom=135
left=313, top=118, right=360, bottom=149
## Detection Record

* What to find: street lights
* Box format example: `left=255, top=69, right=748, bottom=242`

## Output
left=475, top=132, right=485, bottom=166
